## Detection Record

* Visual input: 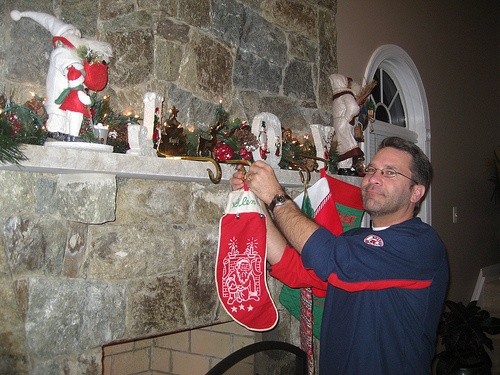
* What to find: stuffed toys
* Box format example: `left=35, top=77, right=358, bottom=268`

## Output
left=10, top=9, right=113, bottom=138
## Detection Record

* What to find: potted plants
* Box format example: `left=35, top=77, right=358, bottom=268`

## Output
left=434, top=299, right=500, bottom=375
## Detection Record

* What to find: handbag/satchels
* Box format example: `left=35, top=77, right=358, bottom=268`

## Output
left=290, top=176, right=346, bottom=299
left=214, top=176, right=280, bottom=332
left=279, top=171, right=367, bottom=342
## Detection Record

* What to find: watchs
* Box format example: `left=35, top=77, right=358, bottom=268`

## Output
left=268, top=194, right=290, bottom=213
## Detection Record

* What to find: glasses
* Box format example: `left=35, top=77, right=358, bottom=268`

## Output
left=364, top=165, right=419, bottom=186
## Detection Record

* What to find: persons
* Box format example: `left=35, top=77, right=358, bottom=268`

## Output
left=229, top=137, right=449, bottom=375
